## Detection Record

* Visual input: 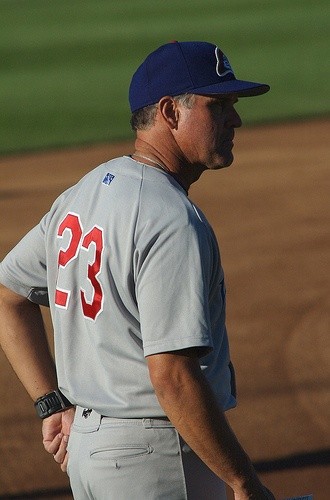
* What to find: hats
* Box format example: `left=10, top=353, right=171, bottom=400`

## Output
left=129, top=39, right=270, bottom=114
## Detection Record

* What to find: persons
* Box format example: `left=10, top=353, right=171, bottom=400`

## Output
left=0, top=40, right=278, bottom=500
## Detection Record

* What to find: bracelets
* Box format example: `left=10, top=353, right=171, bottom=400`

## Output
left=34, top=388, right=74, bottom=419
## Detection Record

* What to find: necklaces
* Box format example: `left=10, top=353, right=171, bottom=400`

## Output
left=130, top=151, right=170, bottom=174
